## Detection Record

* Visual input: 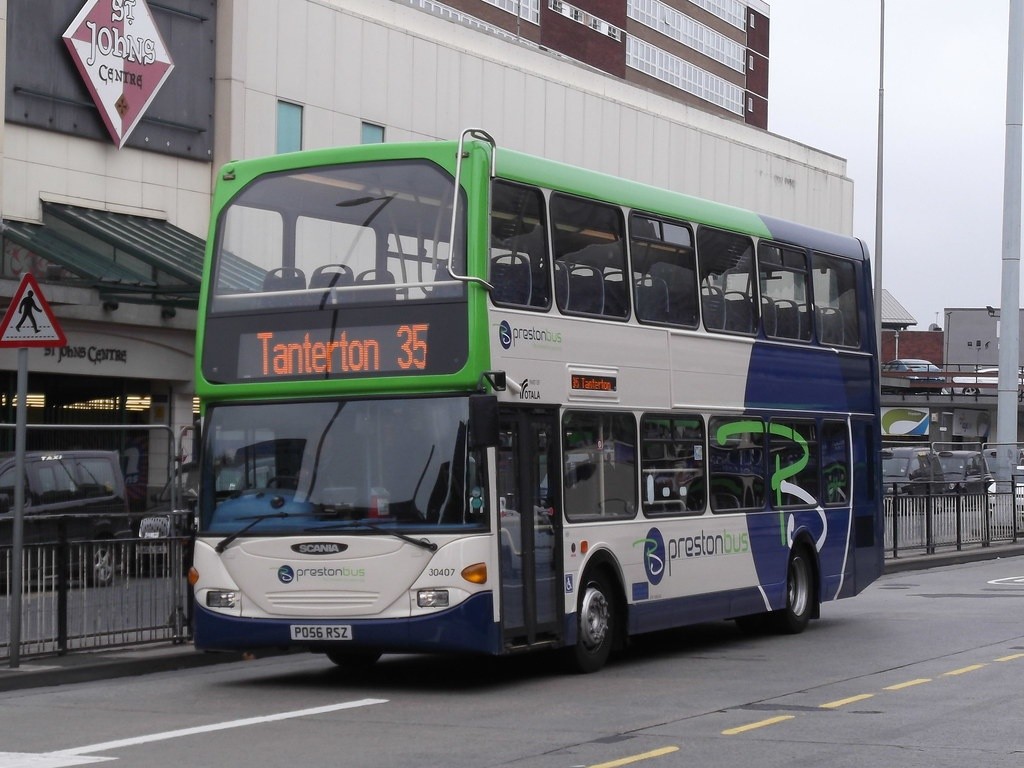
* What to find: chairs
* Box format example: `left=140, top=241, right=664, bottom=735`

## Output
left=635, top=276, right=670, bottom=321
left=567, top=265, right=605, bottom=315
left=820, top=307, right=844, bottom=345
left=489, top=254, right=532, bottom=305
left=432, top=259, right=465, bottom=296
left=680, top=491, right=791, bottom=509
left=675, top=284, right=726, bottom=329
left=798, top=303, right=823, bottom=342
left=531, top=260, right=570, bottom=310
left=774, top=299, right=800, bottom=340
left=725, top=291, right=754, bottom=333
left=750, top=295, right=777, bottom=337
left=355, top=269, right=395, bottom=285
left=602, top=271, right=638, bottom=315
left=308, top=263, right=354, bottom=289
left=264, top=267, right=306, bottom=292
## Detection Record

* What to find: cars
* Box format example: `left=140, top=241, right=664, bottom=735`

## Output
left=882, top=446, right=937, bottom=515
left=984, top=466, right=1024, bottom=521
left=135, top=462, right=198, bottom=578
left=901, top=451, right=997, bottom=519
left=881, top=358, right=945, bottom=395
left=499, top=496, right=556, bottom=579
left=951, top=368, right=1024, bottom=396
left=0, top=450, right=135, bottom=595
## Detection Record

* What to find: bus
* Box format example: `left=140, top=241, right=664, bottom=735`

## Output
left=187, top=128, right=885, bottom=673
